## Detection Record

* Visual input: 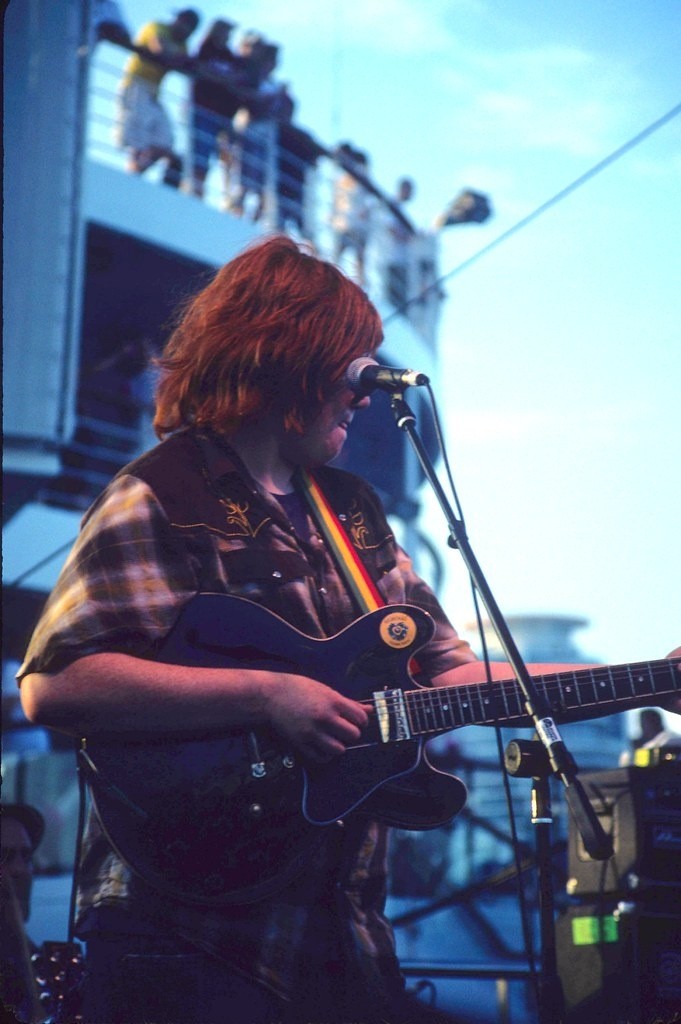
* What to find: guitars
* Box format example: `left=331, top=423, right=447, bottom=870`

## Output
left=81, top=588, right=681, bottom=917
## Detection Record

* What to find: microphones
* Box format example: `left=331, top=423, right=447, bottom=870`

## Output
left=347, top=357, right=429, bottom=397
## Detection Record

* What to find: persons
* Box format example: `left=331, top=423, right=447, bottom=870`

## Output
left=0, top=801, right=48, bottom=1024
left=119, top=8, right=414, bottom=312
left=13, top=238, right=679, bottom=1024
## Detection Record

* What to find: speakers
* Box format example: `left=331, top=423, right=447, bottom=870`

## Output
left=551, top=764, right=681, bottom=1024
